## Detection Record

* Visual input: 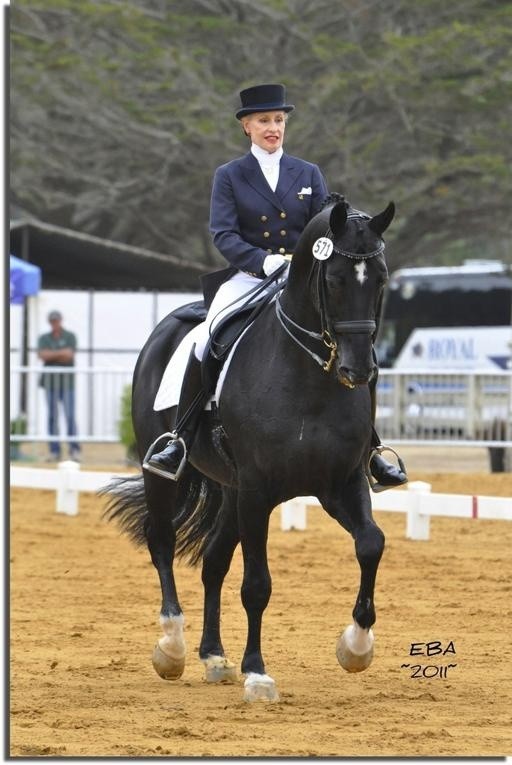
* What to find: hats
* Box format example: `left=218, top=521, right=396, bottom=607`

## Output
left=235, top=84, right=295, bottom=120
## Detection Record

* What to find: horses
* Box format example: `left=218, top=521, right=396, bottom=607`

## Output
left=95, top=191, right=396, bottom=706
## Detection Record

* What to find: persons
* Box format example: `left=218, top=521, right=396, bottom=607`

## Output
left=36, top=311, right=81, bottom=462
left=149, top=85, right=408, bottom=486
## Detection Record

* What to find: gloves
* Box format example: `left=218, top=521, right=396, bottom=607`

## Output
left=262, top=254, right=291, bottom=280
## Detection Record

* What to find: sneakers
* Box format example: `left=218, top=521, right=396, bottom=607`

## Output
left=41, top=447, right=83, bottom=464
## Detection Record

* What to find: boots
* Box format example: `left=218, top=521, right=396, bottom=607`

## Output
left=148, top=342, right=211, bottom=473
left=357, top=388, right=408, bottom=486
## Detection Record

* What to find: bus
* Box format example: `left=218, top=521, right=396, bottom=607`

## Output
left=372, top=259, right=512, bottom=437
